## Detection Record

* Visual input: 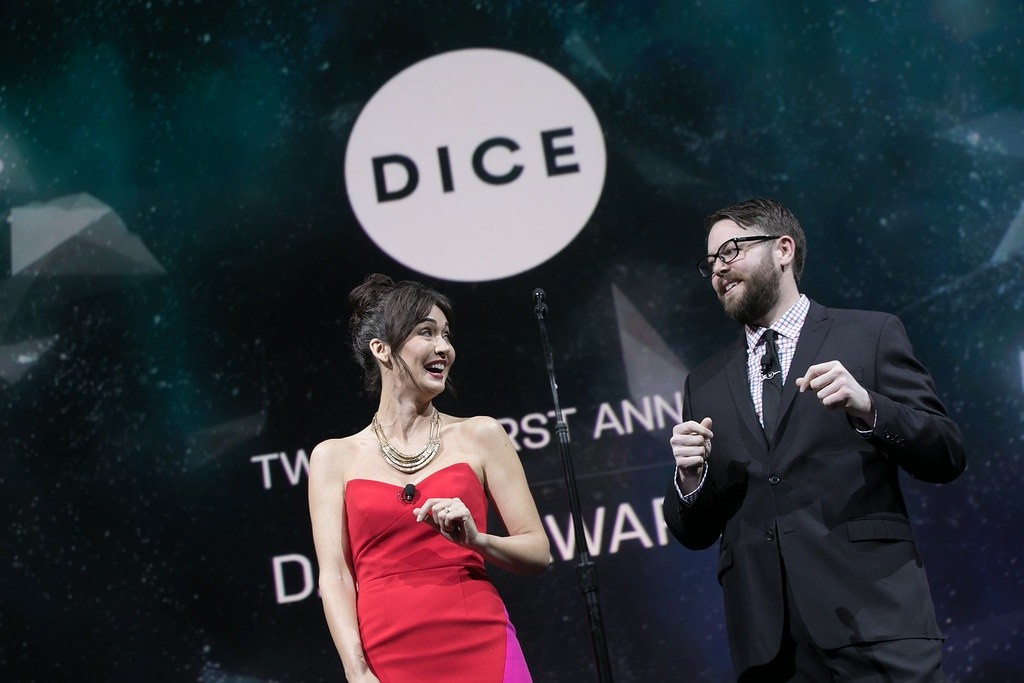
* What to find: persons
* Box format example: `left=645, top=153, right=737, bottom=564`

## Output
left=307, top=273, right=551, bottom=683
left=661, top=200, right=967, bottom=683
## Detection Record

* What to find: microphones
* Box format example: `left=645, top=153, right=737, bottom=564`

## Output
left=532, top=288, right=561, bottom=423
left=759, top=354, right=772, bottom=375
left=399, top=483, right=415, bottom=501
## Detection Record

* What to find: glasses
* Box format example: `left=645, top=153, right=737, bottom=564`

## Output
left=696, top=235, right=781, bottom=278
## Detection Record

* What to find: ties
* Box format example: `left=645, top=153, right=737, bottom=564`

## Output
left=762, top=329, right=785, bottom=450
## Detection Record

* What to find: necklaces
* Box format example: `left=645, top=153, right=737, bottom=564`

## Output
left=372, top=408, right=441, bottom=473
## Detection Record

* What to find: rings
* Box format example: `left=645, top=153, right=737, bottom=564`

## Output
left=445, top=508, right=450, bottom=513
